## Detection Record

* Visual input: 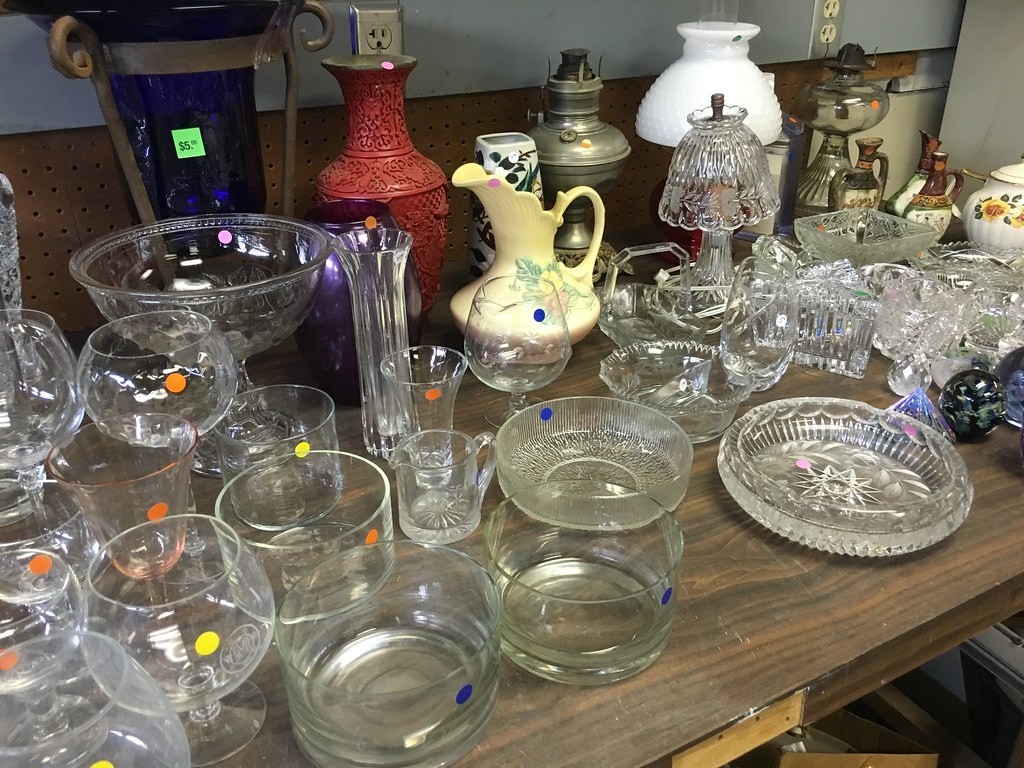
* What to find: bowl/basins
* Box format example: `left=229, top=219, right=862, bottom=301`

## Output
left=599, top=337, right=754, bottom=443
left=795, top=206, right=936, bottom=274
left=494, top=396, right=697, bottom=531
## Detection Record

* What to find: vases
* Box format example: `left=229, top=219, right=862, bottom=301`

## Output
left=634, top=21, right=783, bottom=149
left=329, top=229, right=423, bottom=461
left=314, top=55, right=451, bottom=323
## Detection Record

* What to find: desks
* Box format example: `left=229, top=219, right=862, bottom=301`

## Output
left=0, top=306, right=1024, bottom=768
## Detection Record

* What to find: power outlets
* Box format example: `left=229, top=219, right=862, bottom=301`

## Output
left=807, top=0, right=846, bottom=59
left=349, top=4, right=405, bottom=55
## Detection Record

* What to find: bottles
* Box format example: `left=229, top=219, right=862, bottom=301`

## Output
left=828, top=136, right=890, bottom=214
left=886, top=128, right=963, bottom=243
left=314, top=52, right=449, bottom=318
left=328, top=226, right=422, bottom=456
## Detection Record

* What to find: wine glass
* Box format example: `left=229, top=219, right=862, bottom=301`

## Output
left=0, top=215, right=333, bottom=768
left=459, top=275, right=574, bottom=433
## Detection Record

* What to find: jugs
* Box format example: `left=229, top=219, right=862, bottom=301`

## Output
left=448, top=164, right=609, bottom=364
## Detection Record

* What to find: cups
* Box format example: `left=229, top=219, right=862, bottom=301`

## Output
left=467, top=128, right=548, bottom=268
left=214, top=385, right=344, bottom=533
left=377, top=347, right=469, bottom=472
left=716, top=252, right=803, bottom=391
left=485, top=481, right=685, bottom=690
left=296, top=199, right=420, bottom=406
left=214, top=450, right=395, bottom=626
left=272, top=539, right=501, bottom=768
left=857, top=248, right=1024, bottom=399
left=387, top=430, right=499, bottom=549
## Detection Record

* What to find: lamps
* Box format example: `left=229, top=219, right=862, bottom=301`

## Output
left=792, top=42, right=891, bottom=209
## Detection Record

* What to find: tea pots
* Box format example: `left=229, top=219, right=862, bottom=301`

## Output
left=951, top=154, right=1024, bottom=260
